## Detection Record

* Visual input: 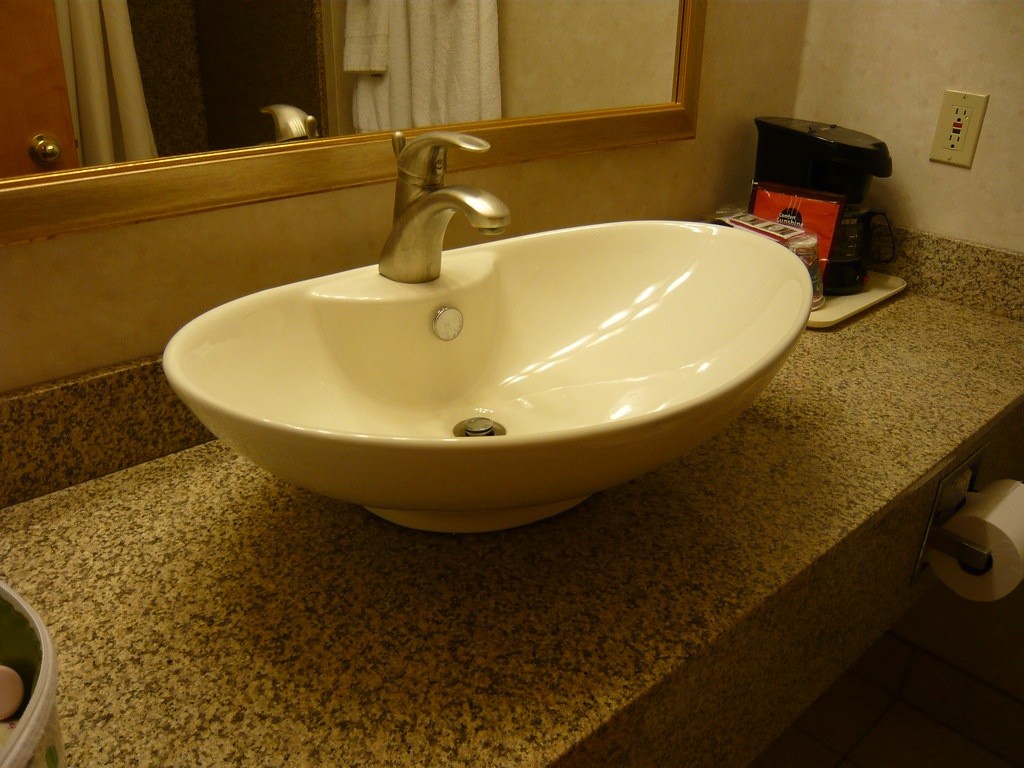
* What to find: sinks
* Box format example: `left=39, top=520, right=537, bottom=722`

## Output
left=159, top=224, right=814, bottom=533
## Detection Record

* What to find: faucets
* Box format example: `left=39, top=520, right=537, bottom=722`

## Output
left=378, top=131, right=511, bottom=283
left=258, top=103, right=319, bottom=143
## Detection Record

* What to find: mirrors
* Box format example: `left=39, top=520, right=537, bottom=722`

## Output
left=0, top=0, right=707, bottom=251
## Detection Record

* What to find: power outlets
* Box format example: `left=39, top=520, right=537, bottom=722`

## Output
left=929, top=89, right=990, bottom=169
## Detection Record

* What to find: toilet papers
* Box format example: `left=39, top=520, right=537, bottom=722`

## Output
left=930, top=479, right=1024, bottom=603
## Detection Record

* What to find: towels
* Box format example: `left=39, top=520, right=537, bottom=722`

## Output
left=341, top=0, right=502, bottom=134
